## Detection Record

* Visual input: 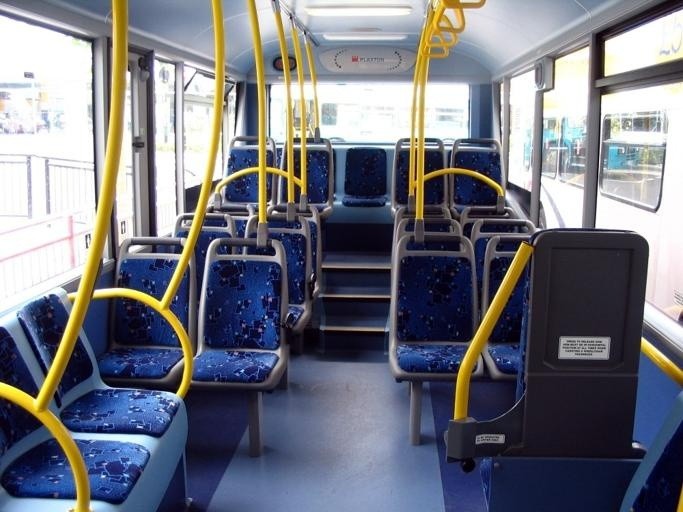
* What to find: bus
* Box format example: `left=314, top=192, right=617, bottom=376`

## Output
left=522, top=111, right=644, bottom=175
left=281, top=83, right=467, bottom=143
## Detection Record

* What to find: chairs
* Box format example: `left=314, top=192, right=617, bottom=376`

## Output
left=341, top=145, right=391, bottom=210
left=389, top=135, right=682, bottom=512
left=107, top=139, right=335, bottom=454
left=0, top=289, right=193, bottom=504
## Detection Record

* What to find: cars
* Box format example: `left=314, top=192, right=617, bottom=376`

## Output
left=0, top=103, right=64, bottom=138
